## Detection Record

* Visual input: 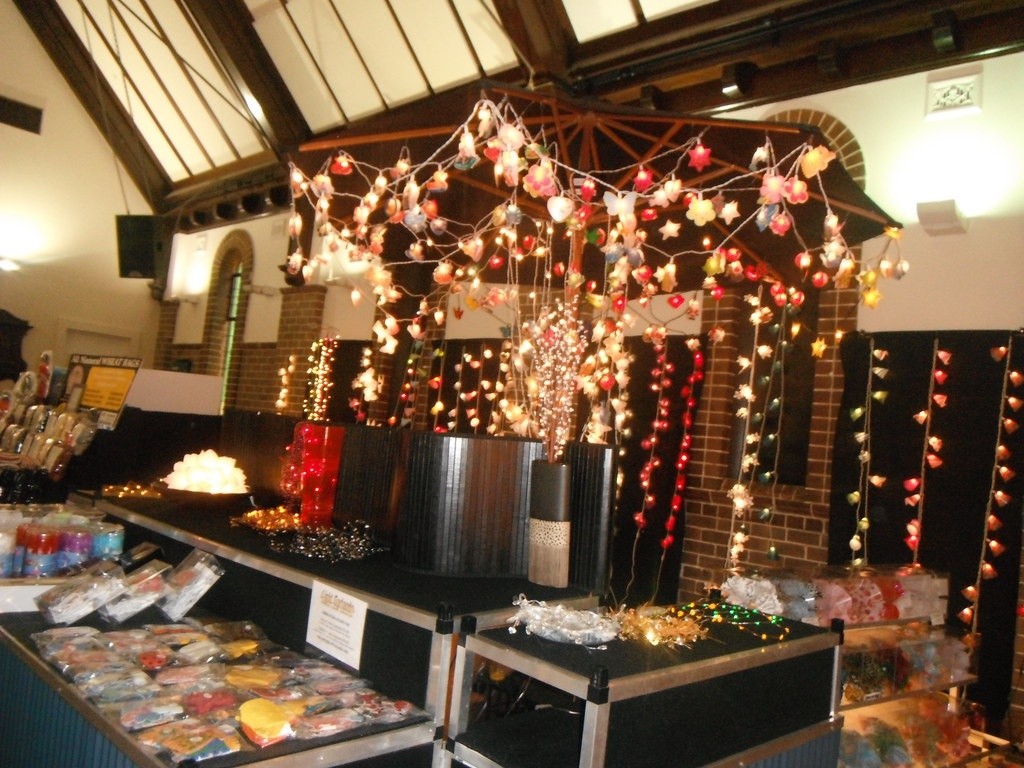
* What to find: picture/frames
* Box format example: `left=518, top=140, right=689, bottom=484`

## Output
left=924, top=70, right=983, bottom=120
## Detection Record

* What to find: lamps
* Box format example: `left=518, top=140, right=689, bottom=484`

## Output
left=917, top=201, right=968, bottom=238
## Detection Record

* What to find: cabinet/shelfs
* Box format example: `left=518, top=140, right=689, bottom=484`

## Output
left=824, top=616, right=1009, bottom=768
left=442, top=587, right=845, bottom=768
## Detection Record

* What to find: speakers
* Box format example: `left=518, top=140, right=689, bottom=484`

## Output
left=114, top=213, right=174, bottom=280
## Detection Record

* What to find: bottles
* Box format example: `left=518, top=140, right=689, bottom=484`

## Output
left=11, top=523, right=125, bottom=575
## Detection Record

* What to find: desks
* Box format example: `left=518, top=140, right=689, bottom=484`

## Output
left=0, top=610, right=433, bottom=768
left=77, top=491, right=600, bottom=768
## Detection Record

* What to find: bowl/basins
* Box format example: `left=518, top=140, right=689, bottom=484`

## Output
left=149, top=480, right=256, bottom=512
